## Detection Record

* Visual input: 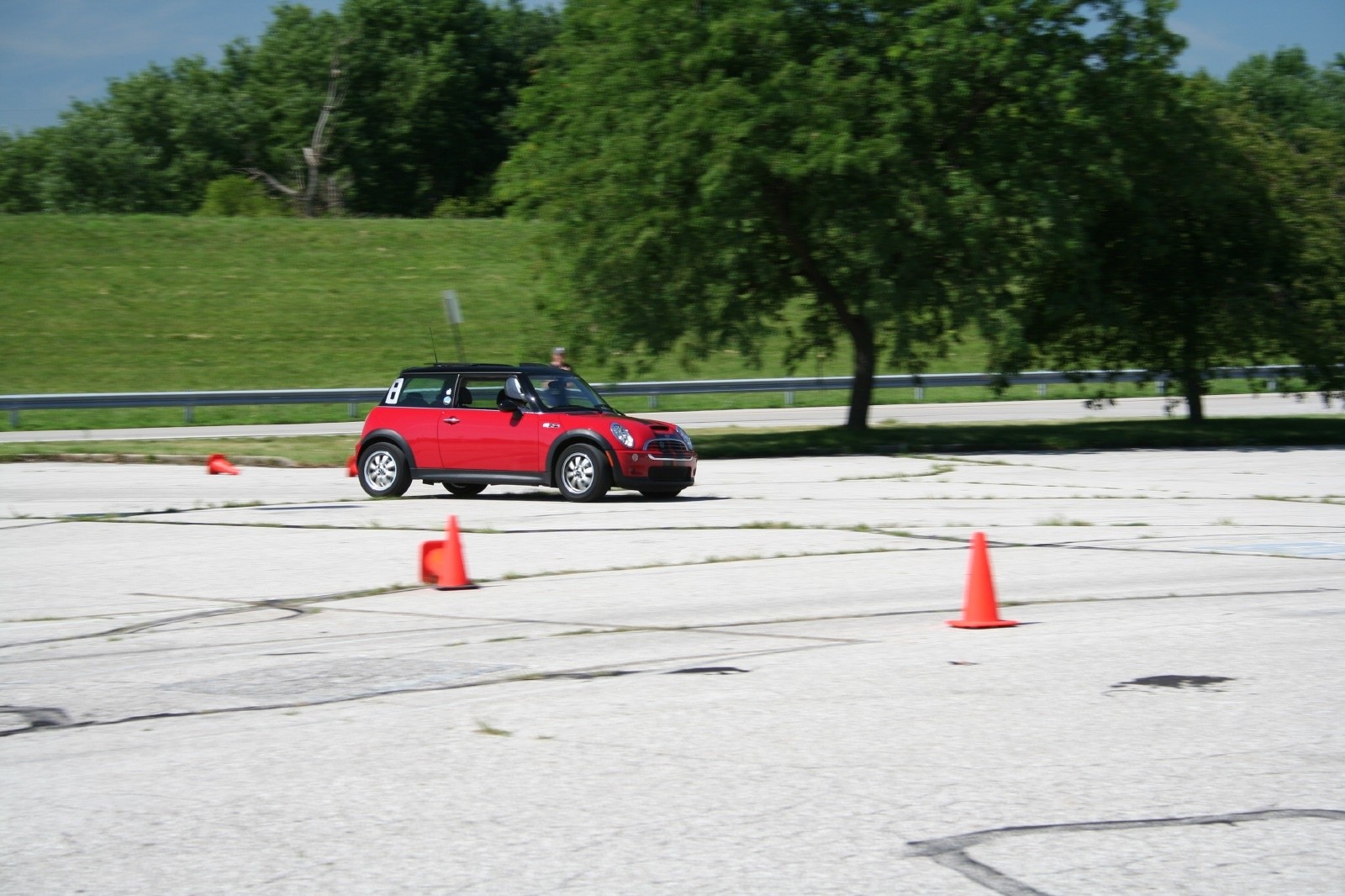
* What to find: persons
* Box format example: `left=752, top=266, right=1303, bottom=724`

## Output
left=544, top=347, right=573, bottom=406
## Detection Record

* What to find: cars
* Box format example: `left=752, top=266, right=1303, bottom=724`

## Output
left=352, top=360, right=699, bottom=501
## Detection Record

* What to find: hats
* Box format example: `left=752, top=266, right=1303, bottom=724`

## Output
left=552, top=347, right=566, bottom=354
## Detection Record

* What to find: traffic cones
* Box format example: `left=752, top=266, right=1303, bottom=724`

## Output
left=416, top=538, right=457, bottom=588
left=344, top=455, right=360, bottom=481
left=947, top=530, right=1022, bottom=630
left=206, top=452, right=241, bottom=475
left=436, top=515, right=484, bottom=590
